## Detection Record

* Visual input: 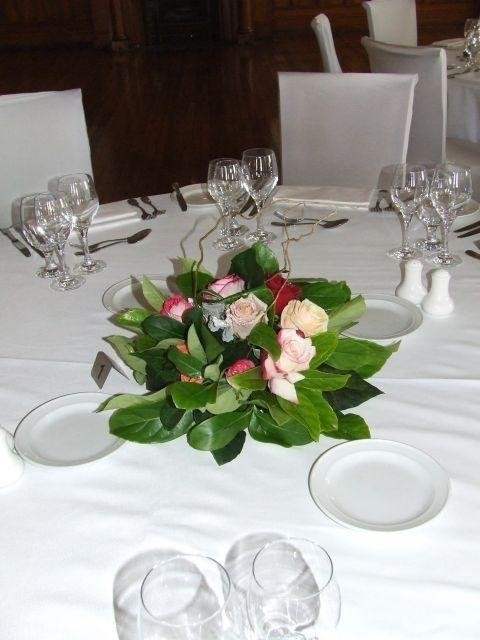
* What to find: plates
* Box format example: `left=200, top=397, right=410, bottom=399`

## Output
left=100, top=273, right=187, bottom=321
left=307, top=437, right=451, bottom=535
left=12, top=389, right=129, bottom=468
left=340, top=293, right=426, bottom=341
left=172, top=182, right=218, bottom=207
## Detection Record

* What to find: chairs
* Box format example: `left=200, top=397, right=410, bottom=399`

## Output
left=277, top=1, right=480, bottom=187
left=0, top=85, right=99, bottom=233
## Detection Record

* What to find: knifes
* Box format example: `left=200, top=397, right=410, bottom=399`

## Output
left=1, top=226, right=31, bottom=259
left=171, top=180, right=188, bottom=212
left=13, top=224, right=46, bottom=260
left=453, top=220, right=480, bottom=260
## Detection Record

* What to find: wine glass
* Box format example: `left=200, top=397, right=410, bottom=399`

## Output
left=56, top=172, right=106, bottom=276
left=430, top=160, right=474, bottom=268
left=240, top=146, right=279, bottom=245
left=206, top=156, right=248, bottom=253
left=413, top=165, right=446, bottom=256
left=461, top=16, right=480, bottom=81
left=33, top=190, right=88, bottom=292
left=213, top=158, right=253, bottom=237
left=385, top=163, right=430, bottom=261
left=19, top=192, right=66, bottom=280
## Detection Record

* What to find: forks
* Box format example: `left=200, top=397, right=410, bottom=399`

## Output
left=367, top=187, right=403, bottom=214
left=127, top=197, right=153, bottom=221
left=140, top=195, right=166, bottom=216
left=73, top=227, right=153, bottom=257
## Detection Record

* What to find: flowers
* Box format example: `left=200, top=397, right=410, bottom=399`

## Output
left=101, top=244, right=401, bottom=467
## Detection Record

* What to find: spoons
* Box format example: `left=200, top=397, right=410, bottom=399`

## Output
left=269, top=208, right=349, bottom=229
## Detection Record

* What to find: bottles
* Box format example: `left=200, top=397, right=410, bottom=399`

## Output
left=421, top=267, right=455, bottom=315
left=394, top=258, right=428, bottom=306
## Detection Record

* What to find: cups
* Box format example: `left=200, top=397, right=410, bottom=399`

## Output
left=136, top=555, right=246, bottom=640
left=245, top=535, right=343, bottom=640
